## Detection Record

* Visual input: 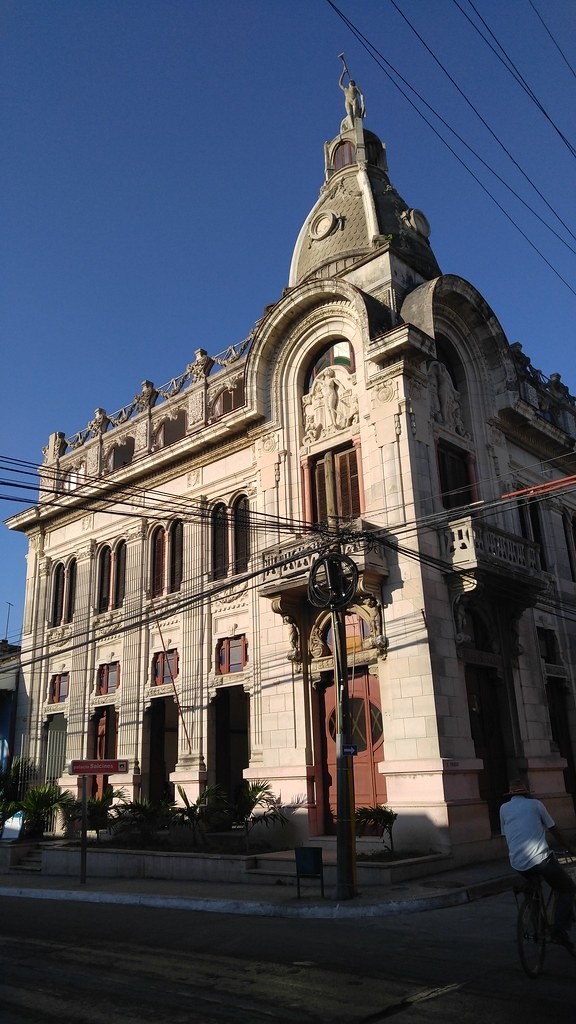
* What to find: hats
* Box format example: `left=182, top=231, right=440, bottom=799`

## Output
left=502, top=779, right=535, bottom=798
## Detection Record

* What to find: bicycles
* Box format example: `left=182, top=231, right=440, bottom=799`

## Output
left=512, top=848, right=576, bottom=979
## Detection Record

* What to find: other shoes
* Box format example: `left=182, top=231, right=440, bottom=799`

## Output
left=552, top=926, right=570, bottom=942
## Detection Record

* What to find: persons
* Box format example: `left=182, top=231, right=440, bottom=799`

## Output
left=436, top=362, right=456, bottom=425
left=339, top=66, right=365, bottom=126
left=498, top=779, right=576, bottom=942
left=311, top=368, right=347, bottom=427
